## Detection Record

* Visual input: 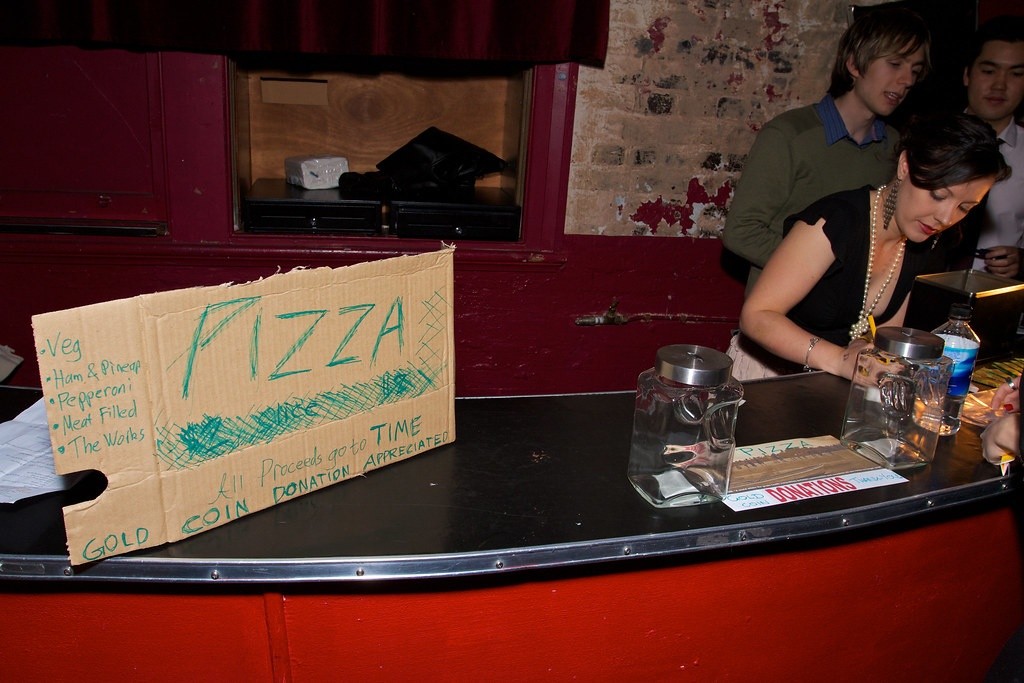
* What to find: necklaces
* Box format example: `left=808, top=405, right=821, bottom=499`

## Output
left=849, top=184, right=908, bottom=340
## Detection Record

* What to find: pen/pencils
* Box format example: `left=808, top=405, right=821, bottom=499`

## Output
left=866, top=314, right=879, bottom=340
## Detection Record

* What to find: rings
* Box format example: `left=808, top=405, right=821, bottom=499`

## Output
left=1005, top=377, right=1016, bottom=390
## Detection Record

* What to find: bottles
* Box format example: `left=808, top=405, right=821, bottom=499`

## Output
left=840, top=326, right=955, bottom=471
left=628, top=344, right=744, bottom=508
left=931, top=304, right=981, bottom=436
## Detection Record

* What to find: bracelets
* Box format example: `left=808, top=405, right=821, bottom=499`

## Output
left=803, top=336, right=823, bottom=373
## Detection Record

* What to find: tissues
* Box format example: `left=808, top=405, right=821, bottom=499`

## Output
left=285, top=154, right=350, bottom=190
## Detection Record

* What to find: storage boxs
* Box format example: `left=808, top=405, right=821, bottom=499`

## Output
left=386, top=187, right=521, bottom=239
left=911, top=270, right=1024, bottom=362
left=244, top=176, right=384, bottom=234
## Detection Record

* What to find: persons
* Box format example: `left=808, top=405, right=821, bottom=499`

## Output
left=722, top=0, right=1024, bottom=302
left=725, top=111, right=1012, bottom=380
left=979, top=368, right=1024, bottom=683
